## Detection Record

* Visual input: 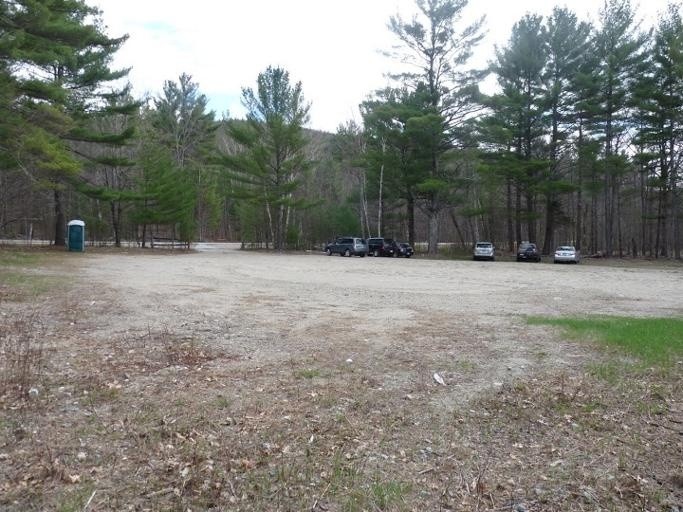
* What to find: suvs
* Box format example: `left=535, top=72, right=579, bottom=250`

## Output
left=517, top=242, right=541, bottom=263
left=323, top=237, right=415, bottom=258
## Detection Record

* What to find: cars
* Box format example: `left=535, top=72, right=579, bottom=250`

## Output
left=553, top=245, right=580, bottom=264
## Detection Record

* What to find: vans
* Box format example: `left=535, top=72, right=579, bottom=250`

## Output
left=472, top=241, right=496, bottom=261
